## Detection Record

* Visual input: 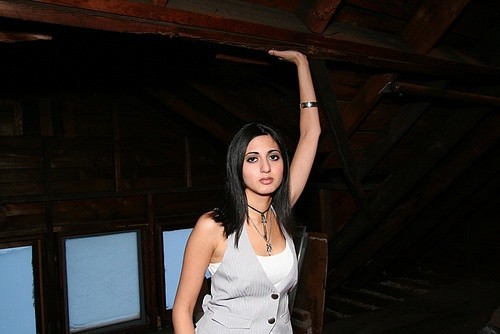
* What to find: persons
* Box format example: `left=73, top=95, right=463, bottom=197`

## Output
left=171, top=48, right=323, bottom=334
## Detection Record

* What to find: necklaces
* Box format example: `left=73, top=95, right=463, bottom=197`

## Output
left=243, top=200, right=272, bottom=240
left=248, top=206, right=273, bottom=256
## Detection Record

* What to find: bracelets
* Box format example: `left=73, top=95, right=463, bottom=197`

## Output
left=300, top=102, right=318, bottom=108
